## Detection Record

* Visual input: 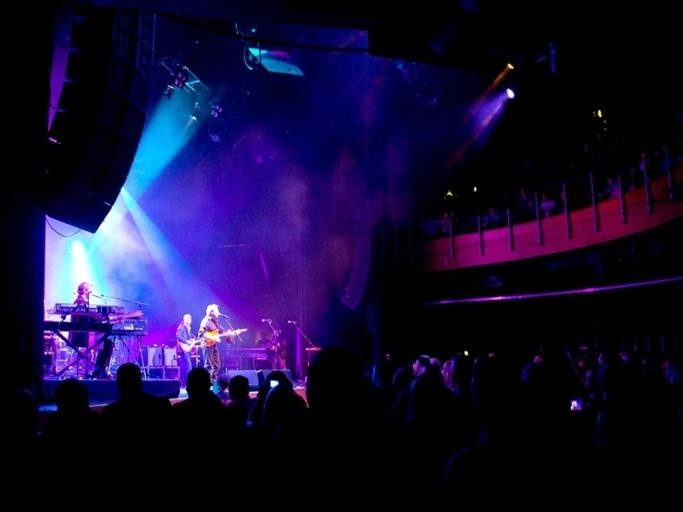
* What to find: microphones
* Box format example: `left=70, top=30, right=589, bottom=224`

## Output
left=262, top=319, right=272, bottom=323
left=218, top=312, right=230, bottom=320
left=91, top=293, right=103, bottom=299
left=287, top=320, right=298, bottom=324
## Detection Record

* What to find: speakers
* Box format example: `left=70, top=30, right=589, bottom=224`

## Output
left=227, top=369, right=292, bottom=391
left=46, top=10, right=156, bottom=233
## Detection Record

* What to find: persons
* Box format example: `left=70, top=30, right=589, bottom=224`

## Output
left=1, top=328, right=682, bottom=512
left=174, top=314, right=200, bottom=389
left=67, top=281, right=114, bottom=379
left=418, top=145, right=679, bottom=239
left=200, top=304, right=233, bottom=387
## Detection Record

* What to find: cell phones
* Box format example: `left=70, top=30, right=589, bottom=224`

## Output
left=569, top=398, right=584, bottom=412
left=269, top=379, right=280, bottom=388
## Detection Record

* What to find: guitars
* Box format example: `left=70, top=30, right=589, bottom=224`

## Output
left=202, top=328, right=247, bottom=347
left=180, top=339, right=201, bottom=353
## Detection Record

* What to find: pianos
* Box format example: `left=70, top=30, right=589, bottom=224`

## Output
left=44, top=319, right=147, bottom=335
left=47, top=303, right=124, bottom=317
